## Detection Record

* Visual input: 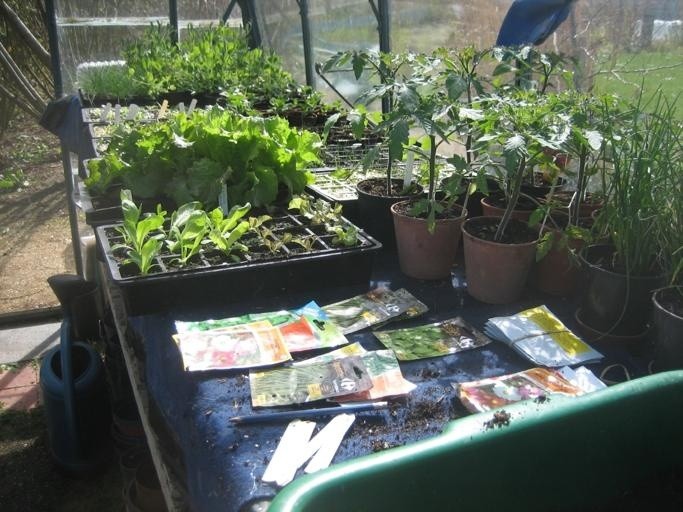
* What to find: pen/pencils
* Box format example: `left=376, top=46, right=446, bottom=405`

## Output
left=227, top=401, right=388, bottom=424
